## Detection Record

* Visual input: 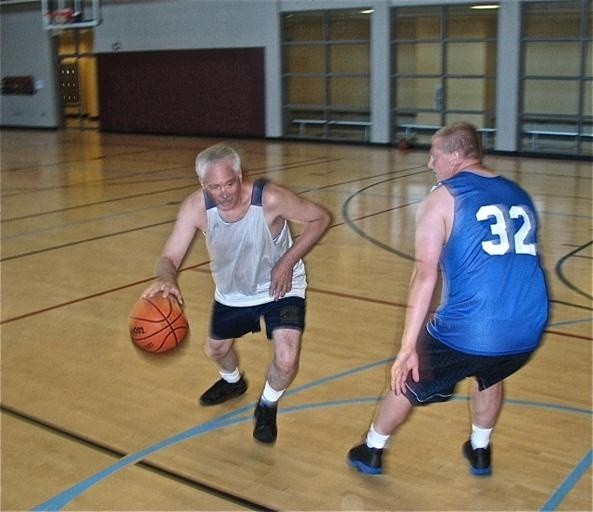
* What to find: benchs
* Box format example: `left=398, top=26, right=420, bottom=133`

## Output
left=291, top=119, right=496, bottom=150
left=519, top=129, right=593, bottom=152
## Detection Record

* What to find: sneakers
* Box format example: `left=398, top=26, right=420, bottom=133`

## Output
left=347, top=442, right=384, bottom=475
left=253, top=396, right=277, bottom=442
left=199, top=373, right=247, bottom=407
left=463, top=439, right=491, bottom=476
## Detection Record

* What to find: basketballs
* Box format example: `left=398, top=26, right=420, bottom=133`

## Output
left=128, top=290, right=189, bottom=353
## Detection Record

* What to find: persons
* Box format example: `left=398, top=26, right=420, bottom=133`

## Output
left=140, top=143, right=331, bottom=446
left=346, top=122, right=550, bottom=477
